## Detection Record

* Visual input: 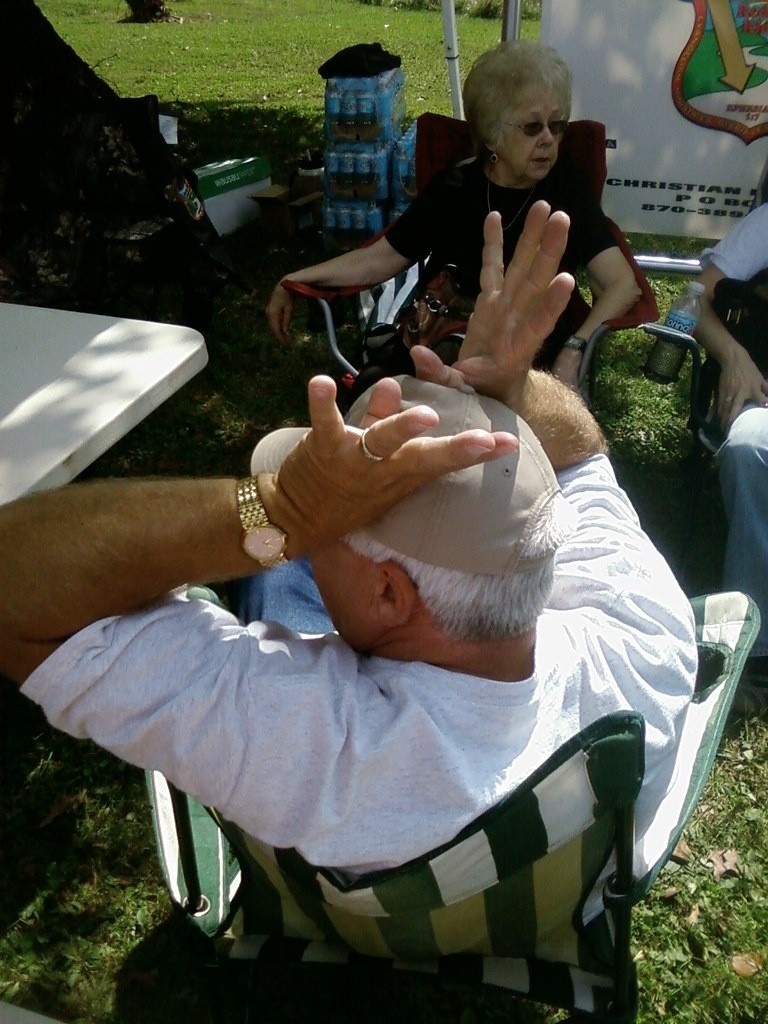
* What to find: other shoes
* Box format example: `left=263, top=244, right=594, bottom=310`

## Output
left=736, top=658, right=768, bottom=715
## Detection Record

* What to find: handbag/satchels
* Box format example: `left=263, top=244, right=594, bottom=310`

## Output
left=702, top=269, right=768, bottom=388
left=398, top=265, right=477, bottom=348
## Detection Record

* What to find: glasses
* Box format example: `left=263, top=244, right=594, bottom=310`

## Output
left=506, top=120, right=570, bottom=136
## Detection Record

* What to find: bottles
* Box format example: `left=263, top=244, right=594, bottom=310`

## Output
left=642, top=282, right=706, bottom=384
left=319, top=64, right=418, bottom=242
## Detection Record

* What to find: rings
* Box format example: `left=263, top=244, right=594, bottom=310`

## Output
left=724, top=396, right=733, bottom=402
left=361, top=429, right=382, bottom=461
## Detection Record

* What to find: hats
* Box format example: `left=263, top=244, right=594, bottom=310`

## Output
left=252, top=374, right=563, bottom=576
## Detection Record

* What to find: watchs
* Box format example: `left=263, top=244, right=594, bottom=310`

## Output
left=564, top=335, right=587, bottom=353
left=235, top=474, right=287, bottom=568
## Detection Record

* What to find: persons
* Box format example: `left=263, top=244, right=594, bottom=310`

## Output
left=0, top=201, right=696, bottom=921
left=685, top=200, right=768, bottom=688
left=267, top=41, right=642, bottom=433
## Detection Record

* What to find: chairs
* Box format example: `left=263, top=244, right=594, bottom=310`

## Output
left=639, top=161, right=767, bottom=699
left=280, top=112, right=660, bottom=407
left=144, top=582, right=761, bottom=1024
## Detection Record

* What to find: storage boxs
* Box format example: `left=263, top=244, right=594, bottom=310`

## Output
left=247, top=174, right=325, bottom=235
left=192, top=154, right=273, bottom=239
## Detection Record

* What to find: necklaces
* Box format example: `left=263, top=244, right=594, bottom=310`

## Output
left=487, top=179, right=536, bottom=230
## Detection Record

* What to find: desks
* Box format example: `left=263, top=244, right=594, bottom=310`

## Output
left=0, top=302, right=209, bottom=508
